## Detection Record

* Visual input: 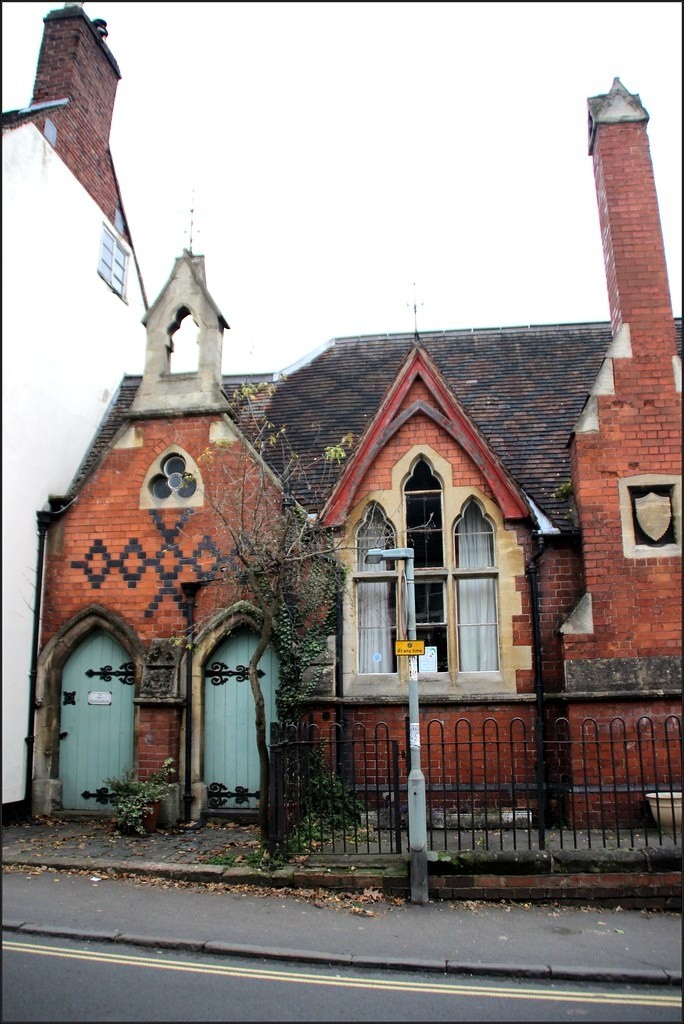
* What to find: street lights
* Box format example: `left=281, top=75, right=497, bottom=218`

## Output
left=364, top=548, right=428, bottom=905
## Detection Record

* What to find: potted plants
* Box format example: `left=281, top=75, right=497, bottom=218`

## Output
left=103, top=758, right=177, bottom=835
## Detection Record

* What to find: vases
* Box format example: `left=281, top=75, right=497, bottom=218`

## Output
left=647, top=793, right=683, bottom=838
